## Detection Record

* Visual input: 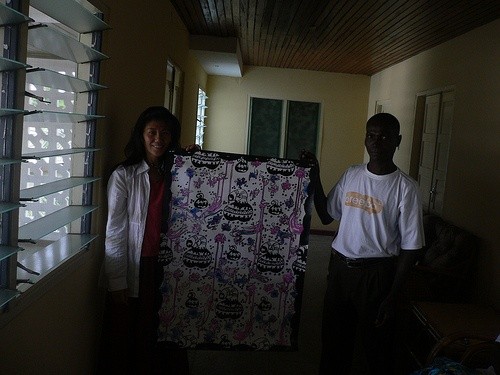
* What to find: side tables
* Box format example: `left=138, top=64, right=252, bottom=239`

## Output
left=404, top=289, right=500, bottom=375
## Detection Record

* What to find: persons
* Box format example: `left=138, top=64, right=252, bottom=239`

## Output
left=98, top=106, right=201, bottom=375
left=299, top=112, right=426, bottom=375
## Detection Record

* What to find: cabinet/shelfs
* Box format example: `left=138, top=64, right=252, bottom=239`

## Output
left=0, top=0, right=106, bottom=309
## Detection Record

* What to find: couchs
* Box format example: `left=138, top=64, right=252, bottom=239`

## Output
left=406, top=208, right=481, bottom=302
